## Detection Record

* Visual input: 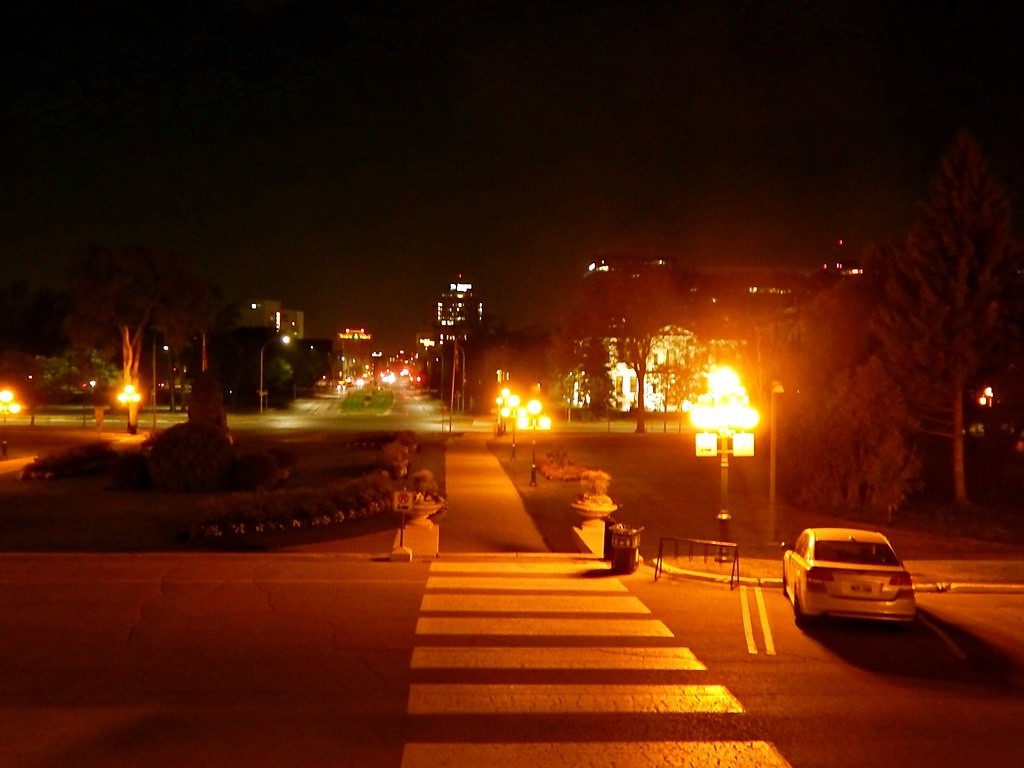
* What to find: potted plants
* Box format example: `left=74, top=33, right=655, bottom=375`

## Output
left=408, top=469, right=445, bottom=515
left=570, top=469, right=618, bottom=518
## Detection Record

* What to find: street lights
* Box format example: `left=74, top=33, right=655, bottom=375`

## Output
left=161, top=345, right=175, bottom=415
left=0, top=390, right=21, bottom=459
left=90, top=380, right=97, bottom=406
left=497, top=388, right=524, bottom=460
left=518, top=400, right=551, bottom=489
left=259, top=337, right=291, bottom=414
left=689, top=366, right=761, bottom=566
left=117, top=384, right=143, bottom=433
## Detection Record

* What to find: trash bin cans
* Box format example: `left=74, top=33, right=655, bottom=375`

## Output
left=609, top=523, right=644, bottom=574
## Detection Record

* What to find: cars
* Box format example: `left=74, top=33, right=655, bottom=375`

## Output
left=780, top=527, right=918, bottom=627
